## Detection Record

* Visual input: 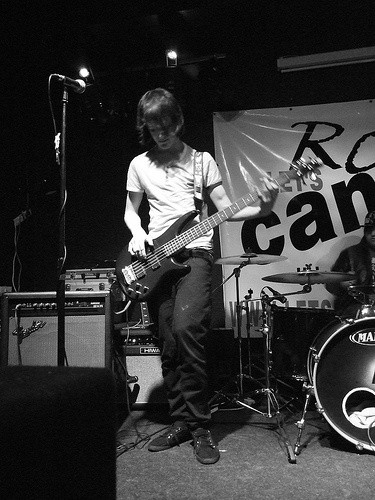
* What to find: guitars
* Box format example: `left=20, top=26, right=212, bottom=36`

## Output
left=115, top=156, right=324, bottom=301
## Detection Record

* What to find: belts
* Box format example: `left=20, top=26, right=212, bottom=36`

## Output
left=178, top=248, right=214, bottom=261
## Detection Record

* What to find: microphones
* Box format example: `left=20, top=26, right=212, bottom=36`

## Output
left=53, top=74, right=86, bottom=94
left=267, top=286, right=287, bottom=303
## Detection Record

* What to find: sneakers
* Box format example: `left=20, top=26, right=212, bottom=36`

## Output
left=192, top=430, right=220, bottom=464
left=148, top=424, right=192, bottom=452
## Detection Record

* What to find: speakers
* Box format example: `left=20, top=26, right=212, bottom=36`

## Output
left=2, top=290, right=165, bottom=410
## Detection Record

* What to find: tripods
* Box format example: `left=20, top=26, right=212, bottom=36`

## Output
left=206, top=261, right=299, bottom=464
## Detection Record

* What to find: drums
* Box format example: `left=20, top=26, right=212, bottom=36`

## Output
left=271, top=305, right=347, bottom=382
left=306, top=303, right=375, bottom=452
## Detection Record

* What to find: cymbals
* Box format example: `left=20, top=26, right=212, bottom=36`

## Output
left=214, top=253, right=288, bottom=266
left=262, top=270, right=359, bottom=287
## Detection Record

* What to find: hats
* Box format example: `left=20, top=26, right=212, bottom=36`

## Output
left=360, top=211, right=375, bottom=227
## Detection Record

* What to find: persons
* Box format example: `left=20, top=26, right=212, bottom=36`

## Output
left=325, top=211, right=375, bottom=318
left=124, top=88, right=279, bottom=464
